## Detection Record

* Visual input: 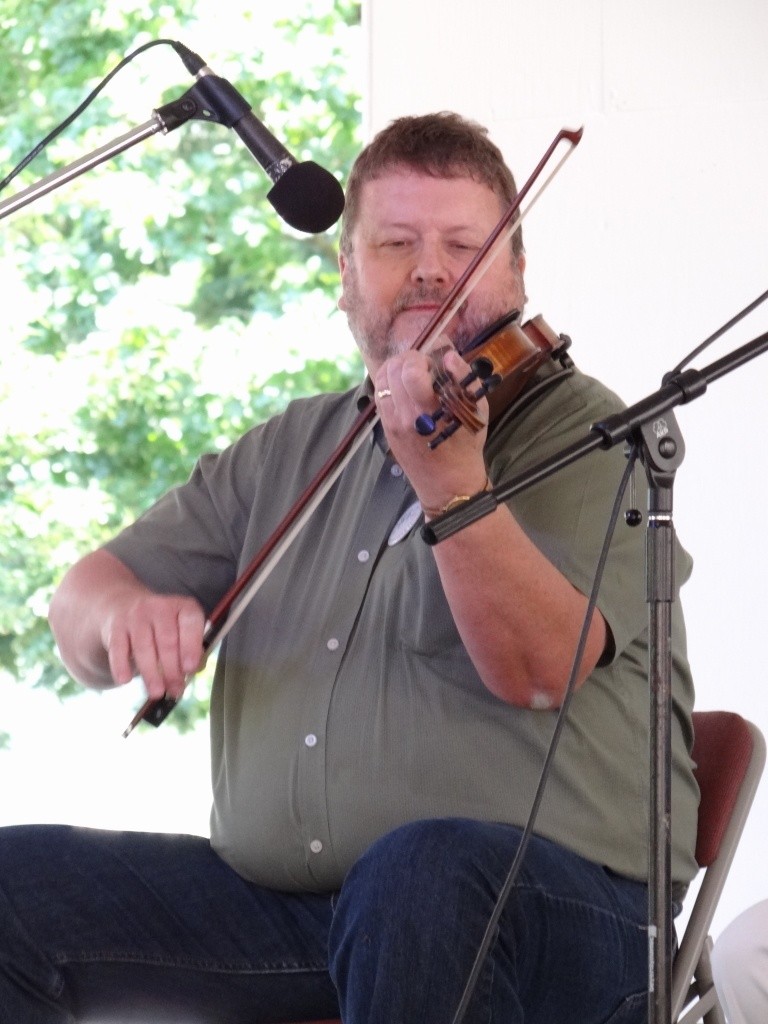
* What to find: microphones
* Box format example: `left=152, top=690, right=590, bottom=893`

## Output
left=174, top=40, right=346, bottom=236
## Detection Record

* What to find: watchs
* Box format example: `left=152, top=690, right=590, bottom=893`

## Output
left=416, top=473, right=495, bottom=526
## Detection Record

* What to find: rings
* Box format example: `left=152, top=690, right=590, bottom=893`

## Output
left=376, top=389, right=392, bottom=399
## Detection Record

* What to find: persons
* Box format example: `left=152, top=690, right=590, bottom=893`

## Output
left=0, top=111, right=702, bottom=1024
left=710, top=899, right=768, bottom=1024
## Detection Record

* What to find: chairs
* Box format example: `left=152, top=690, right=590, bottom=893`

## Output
left=671, top=711, right=767, bottom=1024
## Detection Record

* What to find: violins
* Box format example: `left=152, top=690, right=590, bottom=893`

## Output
left=367, top=311, right=573, bottom=451
